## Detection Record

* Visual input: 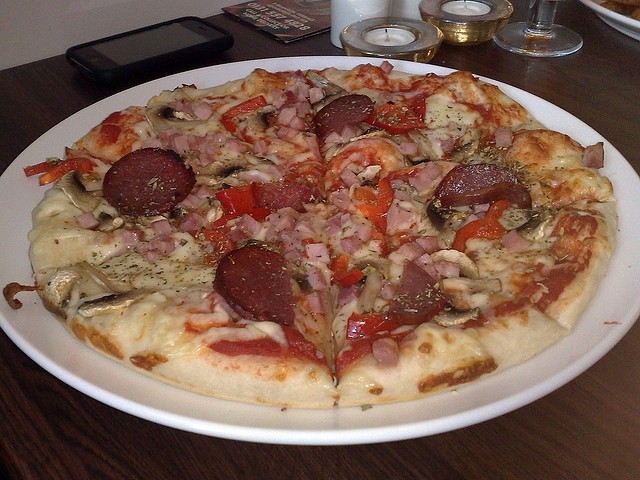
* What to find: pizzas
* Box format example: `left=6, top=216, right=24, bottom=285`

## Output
left=25, top=61, right=619, bottom=408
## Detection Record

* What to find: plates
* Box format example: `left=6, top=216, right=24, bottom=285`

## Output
left=0, top=55, right=640, bottom=448
left=582, top=0, right=640, bottom=43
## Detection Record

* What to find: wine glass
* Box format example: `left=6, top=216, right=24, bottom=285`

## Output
left=492, top=0, right=586, bottom=61
